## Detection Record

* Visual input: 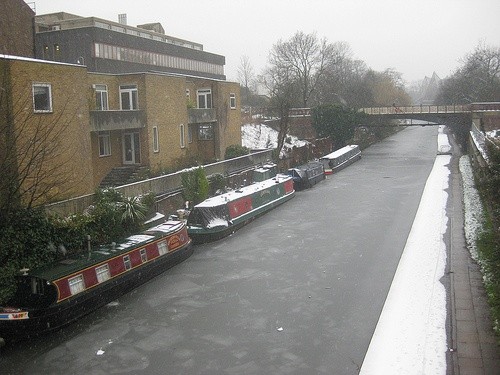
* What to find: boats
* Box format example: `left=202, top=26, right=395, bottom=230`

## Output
left=0, top=217, right=193, bottom=345
left=318, top=141, right=361, bottom=176
left=286, top=161, right=325, bottom=190
left=186, top=174, right=295, bottom=244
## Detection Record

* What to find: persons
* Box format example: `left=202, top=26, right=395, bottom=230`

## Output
left=392, top=102, right=395, bottom=106
